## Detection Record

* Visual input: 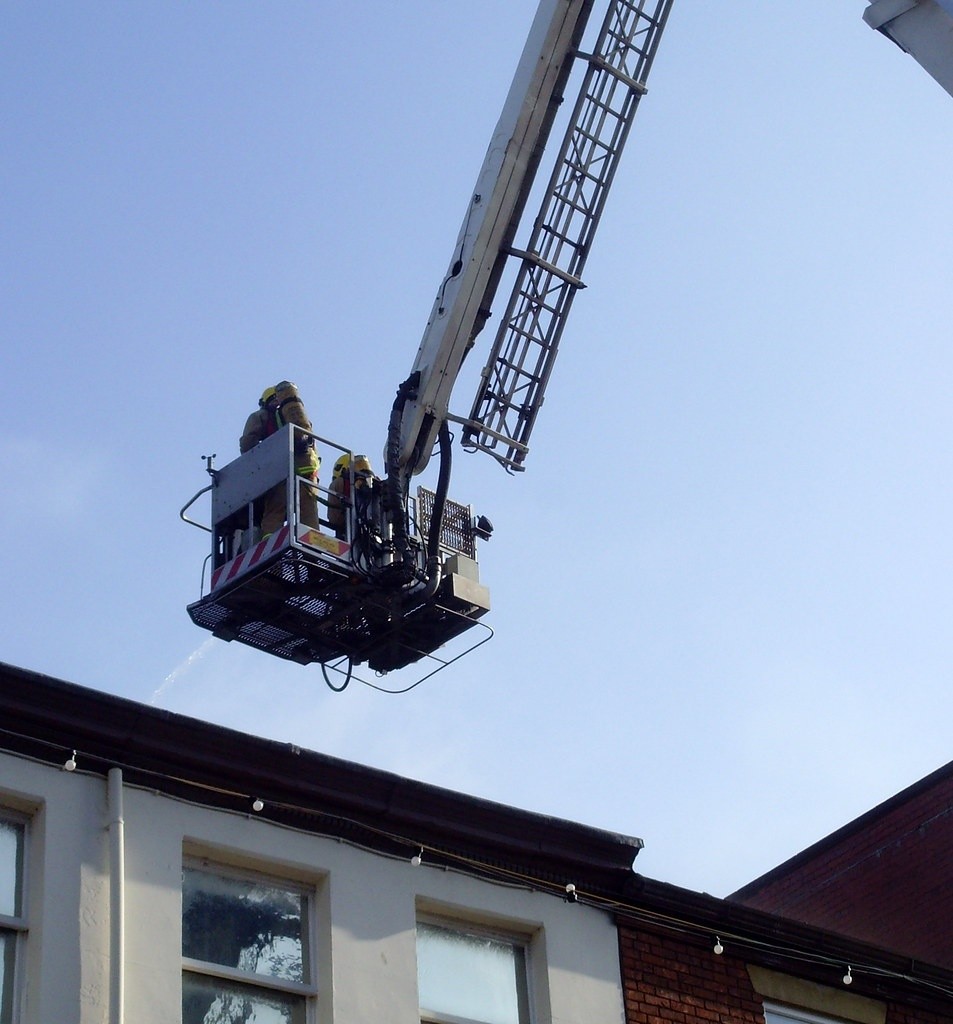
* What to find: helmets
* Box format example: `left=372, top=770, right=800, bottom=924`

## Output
left=262, top=387, right=276, bottom=403
left=333, top=454, right=350, bottom=478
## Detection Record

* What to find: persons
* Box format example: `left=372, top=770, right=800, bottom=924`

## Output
left=328, top=454, right=351, bottom=541
left=240, top=387, right=320, bottom=564
